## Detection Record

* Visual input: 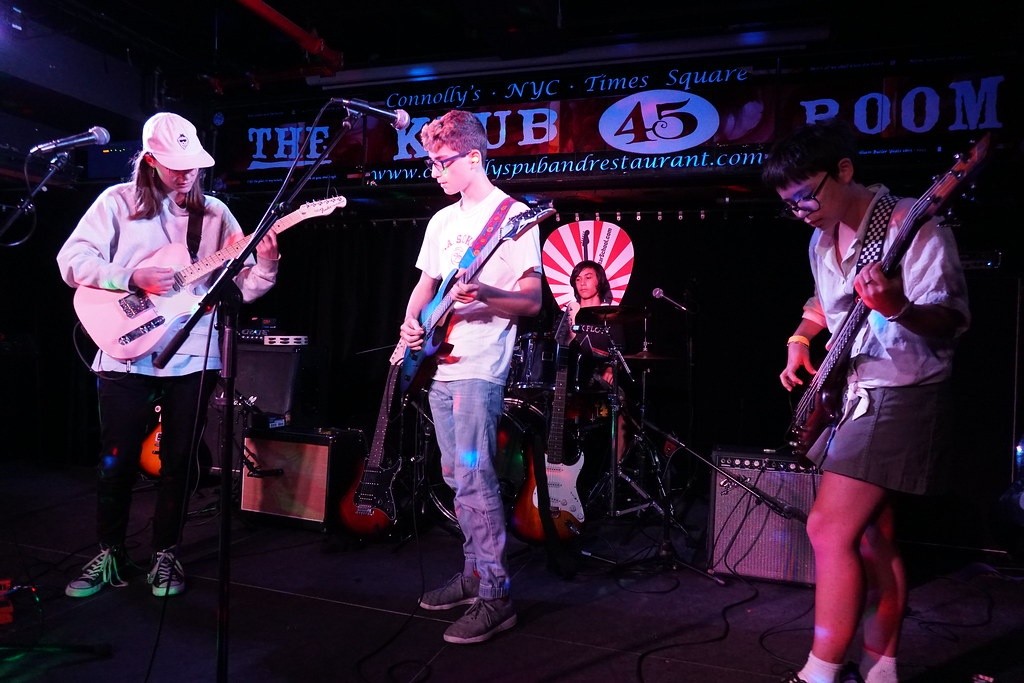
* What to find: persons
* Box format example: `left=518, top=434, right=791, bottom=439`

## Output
left=56, top=111, right=280, bottom=597
left=400, top=109, right=543, bottom=644
left=760, top=127, right=970, bottom=683
left=555, top=261, right=625, bottom=458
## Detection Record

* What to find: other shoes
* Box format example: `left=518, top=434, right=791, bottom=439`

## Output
left=839, top=661, right=865, bottom=683
left=783, top=673, right=805, bottom=683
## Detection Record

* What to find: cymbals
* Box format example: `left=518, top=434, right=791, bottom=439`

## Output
left=623, top=350, right=676, bottom=361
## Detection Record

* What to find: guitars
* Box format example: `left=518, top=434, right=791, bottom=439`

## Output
left=340, top=336, right=406, bottom=537
left=511, top=302, right=589, bottom=545
left=399, top=199, right=557, bottom=391
left=72, top=194, right=348, bottom=365
left=784, top=135, right=989, bottom=473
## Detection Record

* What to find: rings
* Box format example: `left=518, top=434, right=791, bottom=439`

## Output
left=865, top=279, right=872, bottom=285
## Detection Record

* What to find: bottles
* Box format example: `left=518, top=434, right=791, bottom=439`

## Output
left=1015, top=439, right=1024, bottom=473
left=998, top=476, right=1024, bottom=512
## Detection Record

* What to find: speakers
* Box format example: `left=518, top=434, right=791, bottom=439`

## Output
left=707, top=452, right=827, bottom=588
left=201, top=343, right=308, bottom=477
left=240, top=429, right=367, bottom=533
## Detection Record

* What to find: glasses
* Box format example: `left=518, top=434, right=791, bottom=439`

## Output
left=785, top=168, right=838, bottom=220
left=425, top=150, right=471, bottom=171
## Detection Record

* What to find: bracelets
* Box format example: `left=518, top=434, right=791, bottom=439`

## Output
left=787, top=335, right=809, bottom=349
left=887, top=302, right=912, bottom=322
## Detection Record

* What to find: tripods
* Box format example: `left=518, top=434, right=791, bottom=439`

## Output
left=588, top=313, right=785, bottom=584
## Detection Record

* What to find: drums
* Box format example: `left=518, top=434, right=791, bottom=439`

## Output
left=509, top=330, right=557, bottom=391
left=425, top=398, right=553, bottom=525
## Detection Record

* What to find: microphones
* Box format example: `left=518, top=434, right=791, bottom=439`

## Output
left=31, top=126, right=111, bottom=155
left=331, top=98, right=410, bottom=130
left=247, top=469, right=282, bottom=478
left=782, top=505, right=794, bottom=519
left=653, top=288, right=691, bottom=313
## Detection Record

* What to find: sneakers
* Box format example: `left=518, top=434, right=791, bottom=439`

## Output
left=420, top=572, right=482, bottom=611
left=443, top=596, right=518, bottom=644
left=64, top=545, right=129, bottom=598
left=146, top=545, right=187, bottom=596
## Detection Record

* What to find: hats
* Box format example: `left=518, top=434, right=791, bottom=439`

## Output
left=143, top=112, right=215, bottom=169
